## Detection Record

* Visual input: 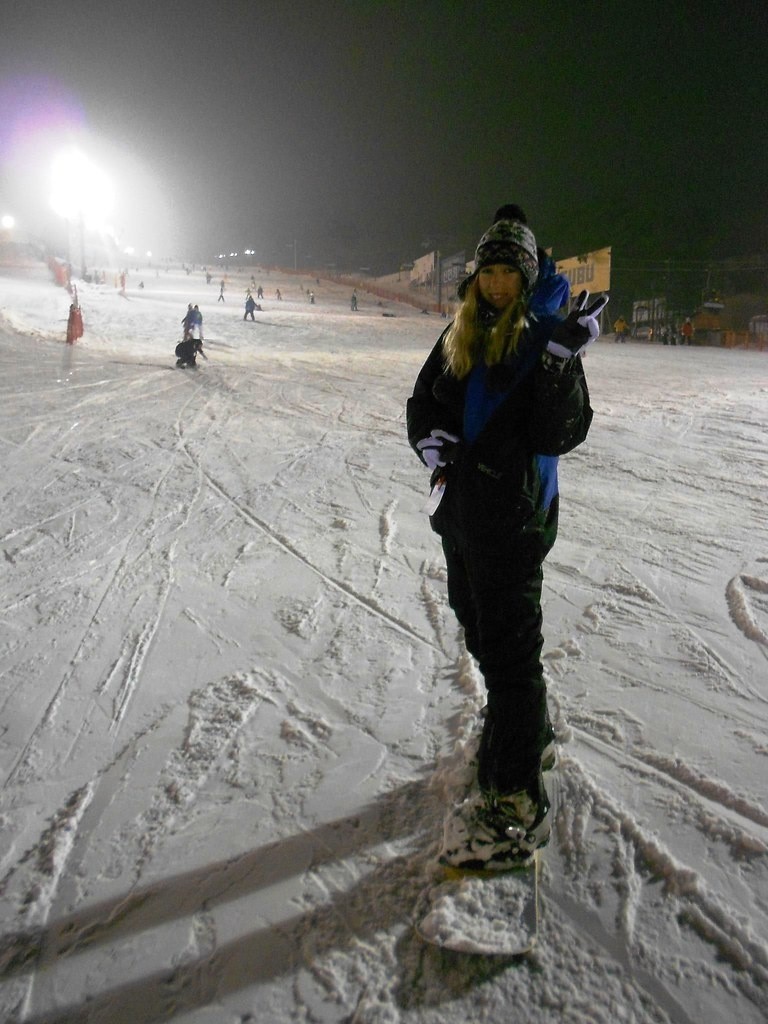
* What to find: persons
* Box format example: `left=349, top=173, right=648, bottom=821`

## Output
left=606, top=299, right=715, bottom=353
left=189, top=305, right=206, bottom=344
left=180, top=302, right=196, bottom=342
left=4, top=231, right=326, bottom=305
left=405, top=201, right=613, bottom=866
left=349, top=289, right=360, bottom=313
left=174, top=337, right=207, bottom=372
left=243, top=295, right=258, bottom=324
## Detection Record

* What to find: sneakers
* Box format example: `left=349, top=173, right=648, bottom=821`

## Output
left=484, top=722, right=562, bottom=793
left=465, top=795, right=552, bottom=871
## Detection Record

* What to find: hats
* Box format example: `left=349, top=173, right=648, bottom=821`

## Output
left=473, top=204, right=538, bottom=292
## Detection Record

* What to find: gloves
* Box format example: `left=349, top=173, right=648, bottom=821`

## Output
left=416, top=430, right=459, bottom=481
left=546, top=291, right=611, bottom=358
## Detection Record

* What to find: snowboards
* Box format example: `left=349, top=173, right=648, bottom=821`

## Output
left=423, top=772, right=541, bottom=957
